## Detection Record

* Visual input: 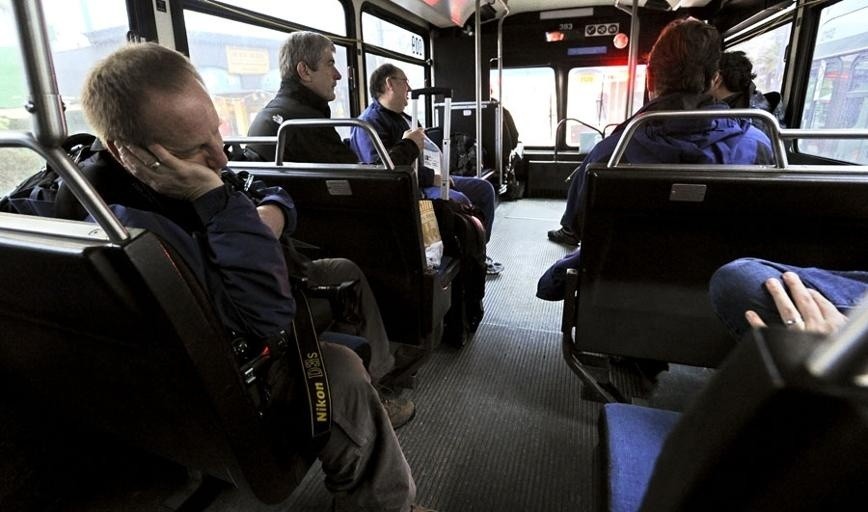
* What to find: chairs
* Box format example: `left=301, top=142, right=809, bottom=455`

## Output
left=437, top=71, right=512, bottom=191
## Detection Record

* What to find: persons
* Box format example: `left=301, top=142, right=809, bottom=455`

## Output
left=79, top=44, right=436, bottom=512
left=248, top=30, right=353, bottom=163
left=351, top=64, right=501, bottom=273
left=536, top=20, right=772, bottom=301
left=491, top=84, right=518, bottom=156
left=548, top=51, right=769, bottom=245
left=708, top=257, right=868, bottom=389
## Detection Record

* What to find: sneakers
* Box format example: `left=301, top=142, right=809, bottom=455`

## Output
left=381, top=399, right=416, bottom=430
left=484, top=256, right=504, bottom=275
left=548, top=227, right=580, bottom=246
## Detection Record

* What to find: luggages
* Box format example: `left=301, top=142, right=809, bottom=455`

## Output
left=422, top=197, right=487, bottom=349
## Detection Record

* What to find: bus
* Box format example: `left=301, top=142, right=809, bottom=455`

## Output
left=760, top=0, right=868, bottom=165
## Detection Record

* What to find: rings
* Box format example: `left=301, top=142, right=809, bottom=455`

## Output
left=786, top=319, right=801, bottom=326
left=150, top=162, right=160, bottom=170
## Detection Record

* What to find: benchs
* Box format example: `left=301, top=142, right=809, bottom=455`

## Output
left=1, top=107, right=326, bottom=512
left=223, top=119, right=456, bottom=379
left=561, top=108, right=867, bottom=404
left=592, top=294, right=868, bottom=512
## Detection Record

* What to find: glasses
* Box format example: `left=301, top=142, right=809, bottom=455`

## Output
left=391, top=78, right=409, bottom=85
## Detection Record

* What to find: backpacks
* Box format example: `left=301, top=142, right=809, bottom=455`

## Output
left=1, top=132, right=271, bottom=416
